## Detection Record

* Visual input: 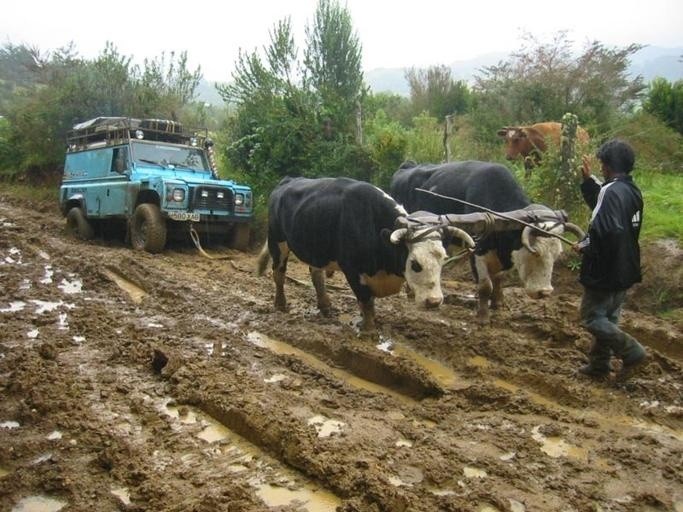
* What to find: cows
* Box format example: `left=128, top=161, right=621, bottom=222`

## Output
left=256, top=174, right=476, bottom=332
left=497, top=121, right=590, bottom=180
left=389, top=157, right=586, bottom=327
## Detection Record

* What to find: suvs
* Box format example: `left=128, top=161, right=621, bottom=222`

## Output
left=57, top=116, right=256, bottom=255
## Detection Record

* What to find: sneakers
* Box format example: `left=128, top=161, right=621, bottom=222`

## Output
left=578, top=363, right=611, bottom=378
left=615, top=351, right=648, bottom=378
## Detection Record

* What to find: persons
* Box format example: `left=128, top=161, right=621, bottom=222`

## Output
left=571, top=140, right=651, bottom=388
